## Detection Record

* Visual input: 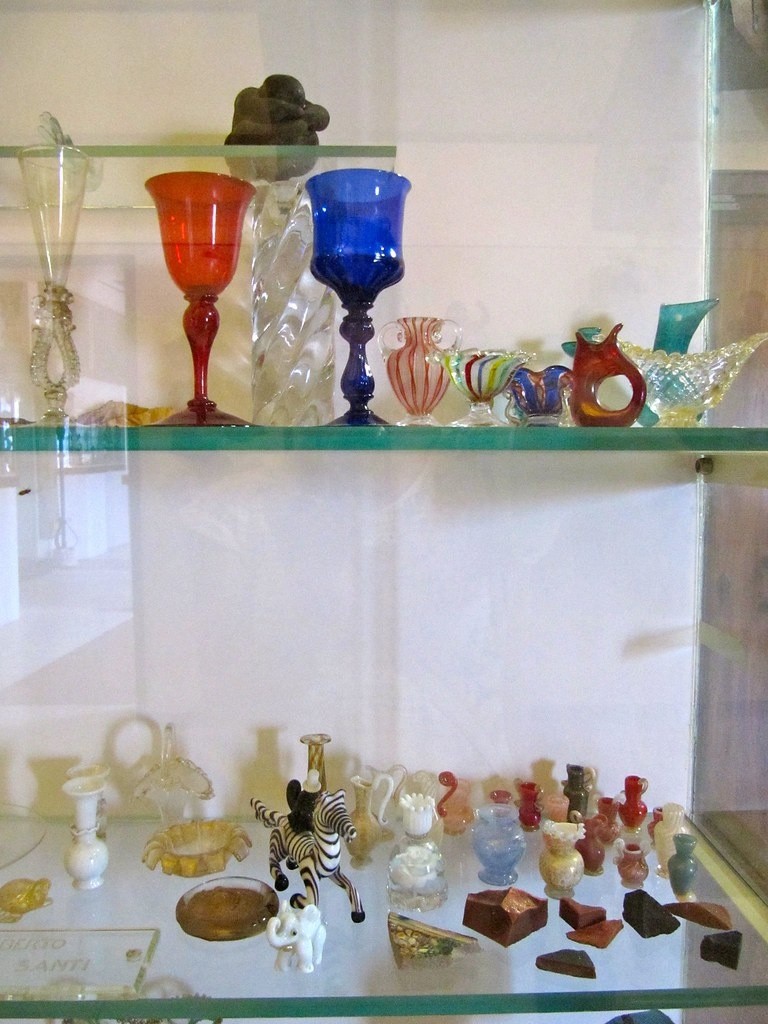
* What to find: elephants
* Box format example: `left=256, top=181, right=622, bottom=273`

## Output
left=266, top=900, right=326, bottom=973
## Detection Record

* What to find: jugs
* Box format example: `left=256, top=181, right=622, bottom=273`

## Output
left=343, top=773, right=395, bottom=869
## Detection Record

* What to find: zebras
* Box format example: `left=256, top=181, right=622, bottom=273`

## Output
left=250, top=789, right=366, bottom=923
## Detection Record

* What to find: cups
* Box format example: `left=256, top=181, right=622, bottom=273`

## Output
left=592, top=333, right=768, bottom=427
left=426, top=344, right=534, bottom=425
left=248, top=178, right=336, bottom=430
left=377, top=315, right=460, bottom=427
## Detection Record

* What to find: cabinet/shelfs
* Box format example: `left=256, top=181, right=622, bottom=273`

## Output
left=0, top=136, right=768, bottom=1024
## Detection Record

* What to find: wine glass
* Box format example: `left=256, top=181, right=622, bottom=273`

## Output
left=140, top=170, right=257, bottom=430
left=12, top=143, right=94, bottom=428
left=307, top=168, right=410, bottom=427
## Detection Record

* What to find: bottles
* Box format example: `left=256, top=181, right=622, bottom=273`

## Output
left=596, top=798, right=621, bottom=842
left=540, top=823, right=585, bottom=901
left=666, top=834, right=698, bottom=903
left=646, top=803, right=689, bottom=880
left=512, top=779, right=543, bottom=832
left=564, top=765, right=587, bottom=819
left=612, top=838, right=651, bottom=890
left=62, top=777, right=108, bottom=892
left=469, top=804, right=525, bottom=887
left=575, top=817, right=604, bottom=877
left=619, top=776, right=650, bottom=834
